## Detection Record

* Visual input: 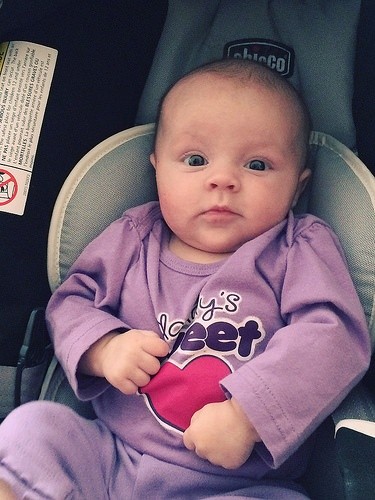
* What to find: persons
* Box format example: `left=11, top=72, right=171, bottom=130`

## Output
left=0, top=57, right=372, bottom=500
